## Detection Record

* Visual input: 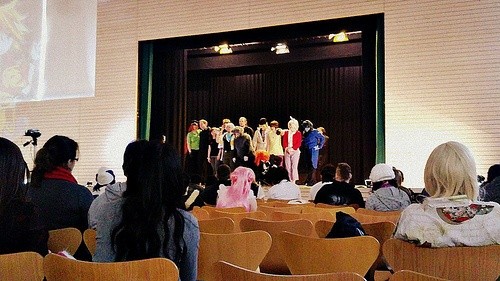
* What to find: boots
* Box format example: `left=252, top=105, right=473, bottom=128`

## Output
left=308, top=168, right=317, bottom=185
left=296, top=171, right=308, bottom=184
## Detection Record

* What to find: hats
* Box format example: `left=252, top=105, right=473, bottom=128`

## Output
left=369, top=163, right=396, bottom=181
left=301, top=119, right=313, bottom=128
left=96, top=166, right=114, bottom=184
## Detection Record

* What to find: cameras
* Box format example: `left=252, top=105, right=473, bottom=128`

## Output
left=24, top=129, right=41, bottom=138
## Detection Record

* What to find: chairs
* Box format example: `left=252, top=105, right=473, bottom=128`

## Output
left=0, top=196, right=500, bottom=281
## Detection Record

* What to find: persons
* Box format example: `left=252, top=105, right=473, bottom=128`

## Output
left=91, top=115, right=499, bottom=213
left=0, top=137, right=49, bottom=281
left=393, top=140, right=500, bottom=247
left=29, top=134, right=95, bottom=262
left=88, top=143, right=200, bottom=280
